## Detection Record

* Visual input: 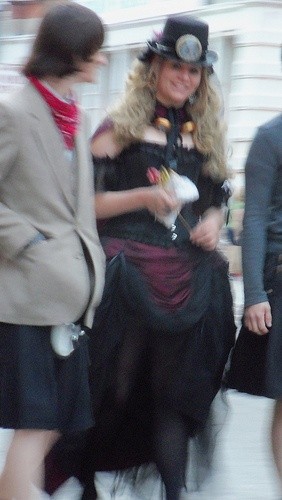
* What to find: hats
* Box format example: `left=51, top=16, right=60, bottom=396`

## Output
left=147, top=13, right=220, bottom=68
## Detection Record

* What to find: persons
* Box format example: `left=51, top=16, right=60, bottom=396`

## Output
left=0, top=3, right=106, bottom=500
left=43, top=15, right=237, bottom=500
left=221, top=113, right=282, bottom=480
